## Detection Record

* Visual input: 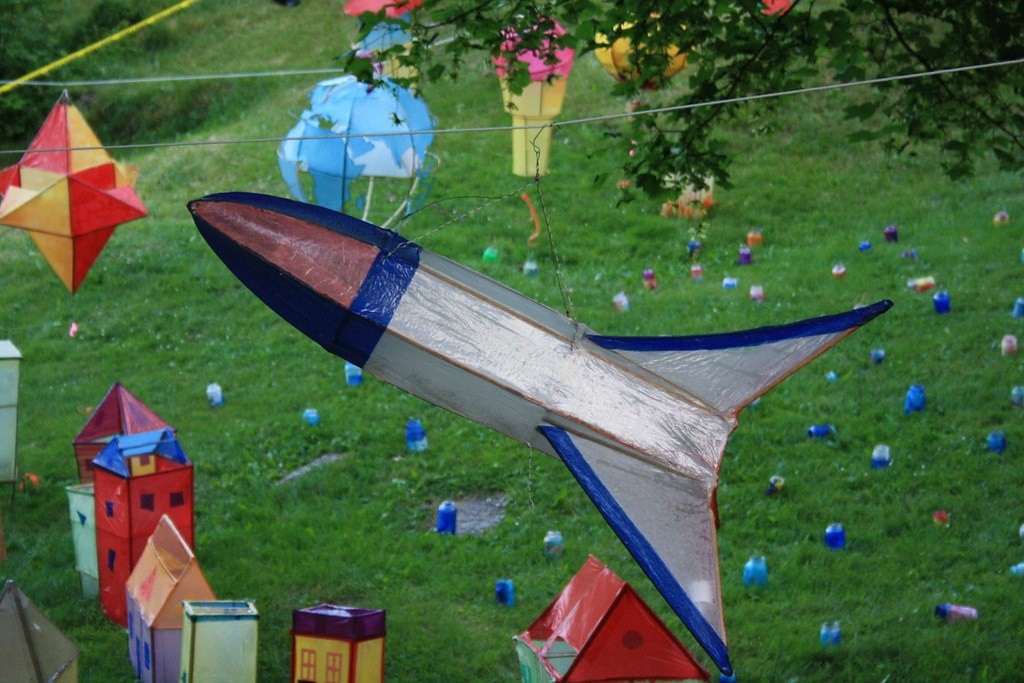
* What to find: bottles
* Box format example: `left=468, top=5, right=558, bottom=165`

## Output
left=436, top=499, right=456, bottom=536
left=932, top=509, right=950, bottom=529
left=820, top=619, right=843, bottom=646
left=473, top=206, right=1024, bottom=467
left=541, top=529, right=564, bottom=563
left=1010, top=562, right=1024, bottom=578
left=824, top=521, right=847, bottom=553
left=303, top=408, right=320, bottom=427
left=404, top=418, right=428, bottom=452
left=933, top=602, right=978, bottom=626
left=495, top=577, right=516, bottom=608
left=766, top=475, right=784, bottom=499
left=742, top=553, right=768, bottom=587
left=342, top=361, right=363, bottom=385
left=206, top=382, right=225, bottom=408
